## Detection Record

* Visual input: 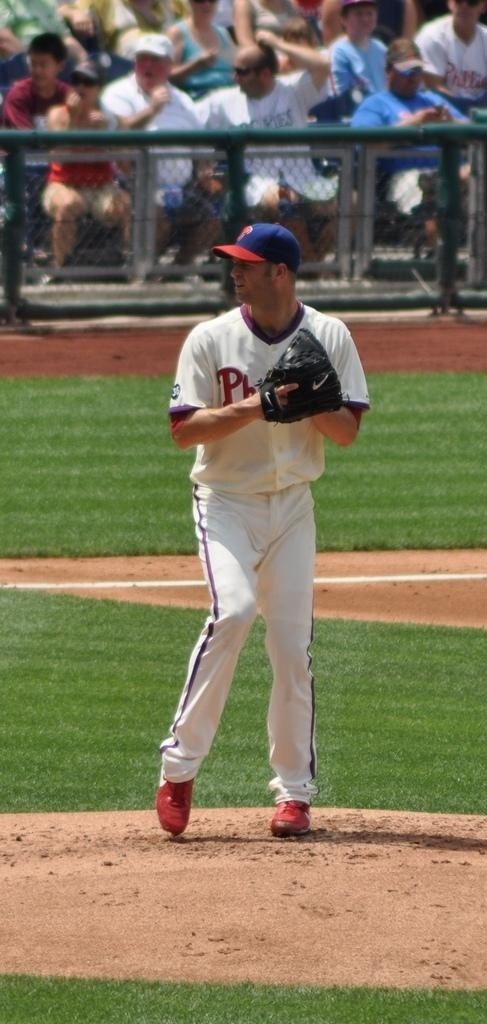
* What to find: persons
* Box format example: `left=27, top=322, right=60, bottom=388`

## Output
left=154, top=219, right=370, bottom=837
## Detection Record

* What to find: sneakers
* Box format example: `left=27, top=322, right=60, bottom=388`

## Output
left=156, top=759, right=193, bottom=836
left=270, top=800, right=311, bottom=837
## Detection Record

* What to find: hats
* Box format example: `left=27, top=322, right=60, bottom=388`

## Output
left=385, top=37, right=426, bottom=73
left=70, top=63, right=101, bottom=83
left=129, top=33, right=175, bottom=67
left=211, top=222, right=302, bottom=275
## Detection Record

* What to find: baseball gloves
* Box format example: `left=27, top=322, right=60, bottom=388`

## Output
left=260, top=330, right=343, bottom=423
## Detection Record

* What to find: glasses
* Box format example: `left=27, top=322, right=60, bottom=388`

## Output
left=452, top=0, right=481, bottom=7
left=232, top=64, right=262, bottom=75
left=390, top=66, right=424, bottom=78
left=71, top=78, right=97, bottom=88
